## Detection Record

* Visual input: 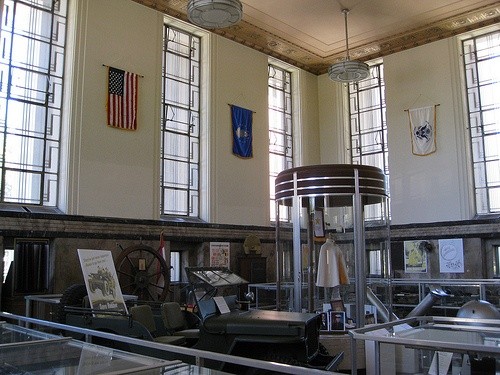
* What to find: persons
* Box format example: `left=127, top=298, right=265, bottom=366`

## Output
left=331, top=314, right=344, bottom=330
left=320, top=313, right=328, bottom=331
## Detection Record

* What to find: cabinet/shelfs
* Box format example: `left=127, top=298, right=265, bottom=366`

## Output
left=238, top=255, right=268, bottom=305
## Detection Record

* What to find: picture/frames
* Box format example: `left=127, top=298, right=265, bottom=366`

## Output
left=404, top=240, right=428, bottom=273
left=77, top=248, right=129, bottom=318
left=329, top=311, right=345, bottom=331
left=316, top=311, right=329, bottom=332
left=330, top=300, right=344, bottom=311
left=439, top=239, right=464, bottom=273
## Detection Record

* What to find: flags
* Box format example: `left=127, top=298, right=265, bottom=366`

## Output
left=156, top=234, right=165, bottom=282
left=409, top=106, right=436, bottom=155
left=230, top=105, right=253, bottom=158
left=107, top=66, right=137, bottom=130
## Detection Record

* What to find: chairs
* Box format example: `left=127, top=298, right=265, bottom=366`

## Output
left=130, top=305, right=186, bottom=347
left=161, top=301, right=199, bottom=338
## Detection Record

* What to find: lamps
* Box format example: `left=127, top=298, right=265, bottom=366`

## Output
left=186, top=0, right=243, bottom=30
left=328, top=8, right=369, bottom=83
